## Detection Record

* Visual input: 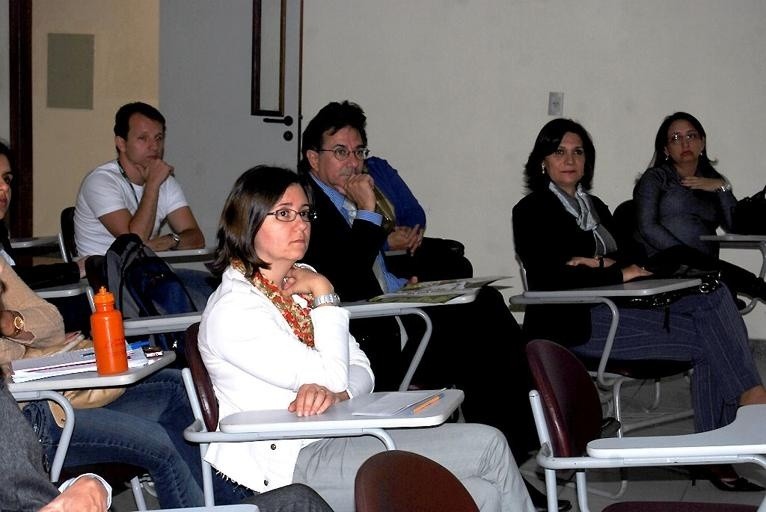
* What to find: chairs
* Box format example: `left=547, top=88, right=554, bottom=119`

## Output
left=7, top=348, right=177, bottom=510
left=612, top=200, right=757, bottom=412
left=524, top=338, right=765, bottom=512
left=182, top=307, right=466, bottom=511
left=56, top=206, right=217, bottom=273
left=510, top=249, right=722, bottom=501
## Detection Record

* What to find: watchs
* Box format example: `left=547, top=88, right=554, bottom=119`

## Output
left=310, top=292, right=341, bottom=306
left=168, top=232, right=181, bottom=249
left=715, top=182, right=731, bottom=192
left=592, top=254, right=605, bottom=269
left=6, top=309, right=25, bottom=339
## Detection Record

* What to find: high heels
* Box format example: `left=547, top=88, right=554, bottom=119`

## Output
left=687, top=464, right=766, bottom=495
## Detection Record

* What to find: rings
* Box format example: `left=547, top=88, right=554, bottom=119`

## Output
left=642, top=267, right=645, bottom=270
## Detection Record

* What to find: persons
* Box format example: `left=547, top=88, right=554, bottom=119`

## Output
left=74, top=101, right=205, bottom=258
left=320, top=101, right=475, bottom=281
left=197, top=165, right=535, bottom=511
left=303, top=112, right=572, bottom=512
left=512, top=119, right=765, bottom=434
left=0, top=139, right=256, bottom=511
left=636, top=111, right=739, bottom=278
left=1, top=360, right=333, bottom=512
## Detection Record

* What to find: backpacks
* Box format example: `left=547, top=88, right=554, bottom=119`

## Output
left=92, top=232, right=199, bottom=360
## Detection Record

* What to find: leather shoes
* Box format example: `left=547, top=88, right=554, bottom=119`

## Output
left=521, top=473, right=573, bottom=512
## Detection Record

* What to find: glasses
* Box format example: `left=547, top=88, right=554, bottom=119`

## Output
left=131, top=133, right=165, bottom=145
left=318, top=146, right=371, bottom=162
left=666, top=133, right=701, bottom=145
left=264, top=207, right=319, bottom=222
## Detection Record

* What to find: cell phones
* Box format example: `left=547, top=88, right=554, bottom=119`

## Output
left=143, top=346, right=163, bottom=357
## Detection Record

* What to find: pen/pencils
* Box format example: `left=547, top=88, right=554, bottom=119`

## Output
left=410, top=392, right=445, bottom=415
left=79, top=340, right=150, bottom=358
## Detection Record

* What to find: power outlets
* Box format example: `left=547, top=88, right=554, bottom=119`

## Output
left=547, top=92, right=564, bottom=116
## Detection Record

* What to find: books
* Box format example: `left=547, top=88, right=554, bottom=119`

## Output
left=367, top=275, right=511, bottom=303
left=8, top=339, right=163, bottom=384
left=351, top=388, right=449, bottom=417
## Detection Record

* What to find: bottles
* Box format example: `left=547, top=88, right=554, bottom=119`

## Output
left=88, top=285, right=128, bottom=375
